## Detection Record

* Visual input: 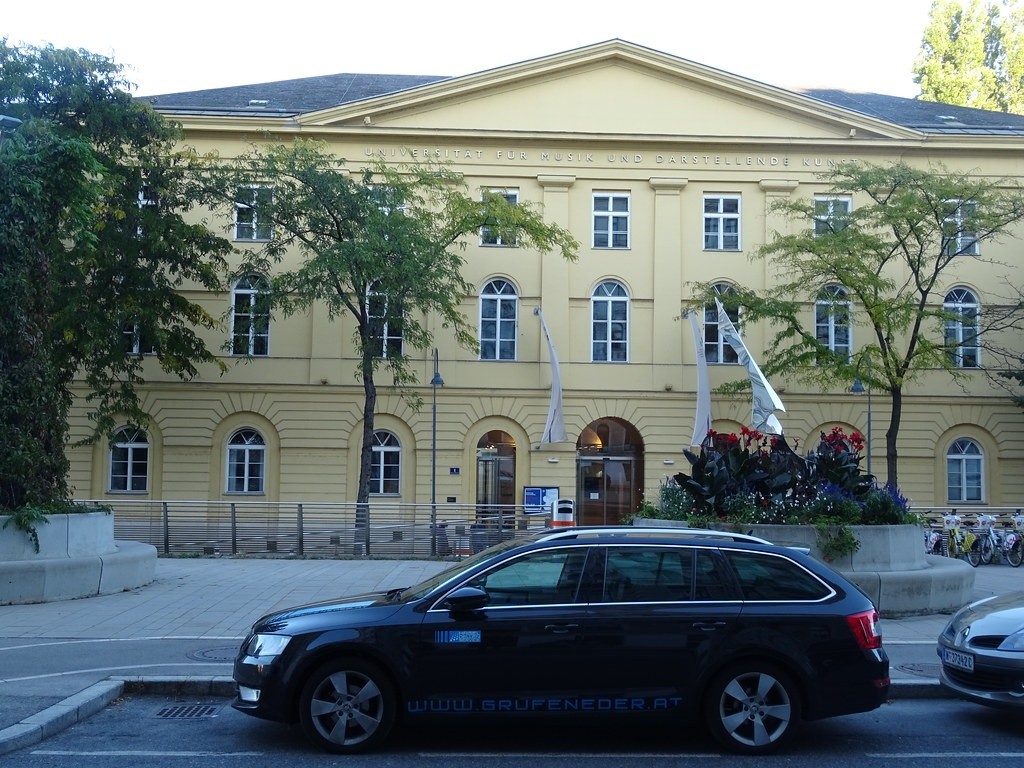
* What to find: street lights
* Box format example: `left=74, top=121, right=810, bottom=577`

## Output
left=428, top=347, right=445, bottom=557
left=849, top=356, right=872, bottom=475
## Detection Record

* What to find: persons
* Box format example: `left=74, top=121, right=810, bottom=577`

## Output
left=471, top=516, right=511, bottom=555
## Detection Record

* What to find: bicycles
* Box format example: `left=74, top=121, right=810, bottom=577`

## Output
left=939, top=512, right=982, bottom=568
left=976, top=513, right=1024, bottom=568
left=918, top=510, right=943, bottom=556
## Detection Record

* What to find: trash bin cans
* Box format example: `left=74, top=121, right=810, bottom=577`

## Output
left=550, top=498, right=576, bottom=530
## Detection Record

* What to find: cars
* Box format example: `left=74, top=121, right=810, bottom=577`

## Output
left=936, top=589, right=1024, bottom=713
left=232, top=525, right=889, bottom=754
